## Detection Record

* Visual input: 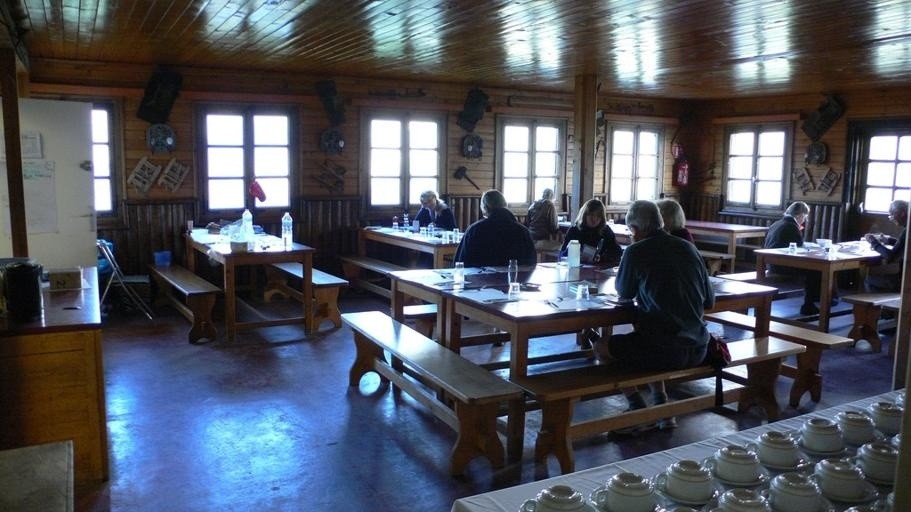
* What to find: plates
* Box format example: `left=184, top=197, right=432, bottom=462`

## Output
left=462, top=134, right=482, bottom=159
left=649, top=473, right=726, bottom=506
left=320, top=128, right=345, bottom=155
left=795, top=439, right=853, bottom=457
left=148, top=124, right=176, bottom=154
left=759, top=452, right=813, bottom=470
left=828, top=482, right=880, bottom=504
left=586, top=490, right=668, bottom=512
left=701, top=456, right=773, bottom=486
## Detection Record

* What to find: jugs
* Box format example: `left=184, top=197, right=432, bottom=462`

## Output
left=703, top=442, right=762, bottom=482
left=868, top=400, right=905, bottom=435
left=752, top=430, right=798, bottom=466
left=848, top=439, right=899, bottom=487
left=791, top=418, right=847, bottom=452
left=807, top=459, right=867, bottom=499
left=588, top=473, right=659, bottom=511
left=517, top=485, right=586, bottom=512
left=655, top=459, right=714, bottom=502
left=709, top=489, right=773, bottom=512
left=833, top=411, right=876, bottom=446
left=759, top=472, right=826, bottom=512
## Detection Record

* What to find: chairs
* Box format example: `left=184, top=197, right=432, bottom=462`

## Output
left=96, top=239, right=155, bottom=320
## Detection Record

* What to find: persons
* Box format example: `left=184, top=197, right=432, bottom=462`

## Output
left=764, top=201, right=838, bottom=316
left=660, top=197, right=693, bottom=242
left=527, top=188, right=558, bottom=241
left=415, top=191, right=457, bottom=268
left=864, top=200, right=907, bottom=293
left=596, top=200, right=715, bottom=433
left=455, top=190, right=537, bottom=345
left=560, top=198, right=623, bottom=264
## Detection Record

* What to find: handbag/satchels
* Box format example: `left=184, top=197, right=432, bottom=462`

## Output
left=708, top=337, right=733, bottom=369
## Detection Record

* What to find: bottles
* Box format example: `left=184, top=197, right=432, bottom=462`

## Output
left=281, top=211, right=294, bottom=251
left=239, top=208, right=255, bottom=250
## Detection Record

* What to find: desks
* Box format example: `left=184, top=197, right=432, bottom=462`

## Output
left=0, top=265, right=109, bottom=483
left=0, top=439, right=74, bottom=512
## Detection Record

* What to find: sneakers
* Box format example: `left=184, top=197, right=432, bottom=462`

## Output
left=585, top=327, right=600, bottom=342
left=577, top=332, right=592, bottom=350
left=800, top=303, right=819, bottom=315
left=613, top=410, right=658, bottom=437
left=658, top=416, right=679, bottom=429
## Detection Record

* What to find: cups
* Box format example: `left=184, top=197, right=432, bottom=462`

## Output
left=508, top=259, right=519, bottom=285
left=508, top=283, right=521, bottom=301
left=389, top=217, right=462, bottom=243
left=224, top=237, right=248, bottom=258
left=785, top=235, right=890, bottom=258
left=455, top=261, right=465, bottom=284
left=425, top=216, right=425, bottom=217
left=576, top=282, right=589, bottom=301
left=568, top=239, right=582, bottom=267
